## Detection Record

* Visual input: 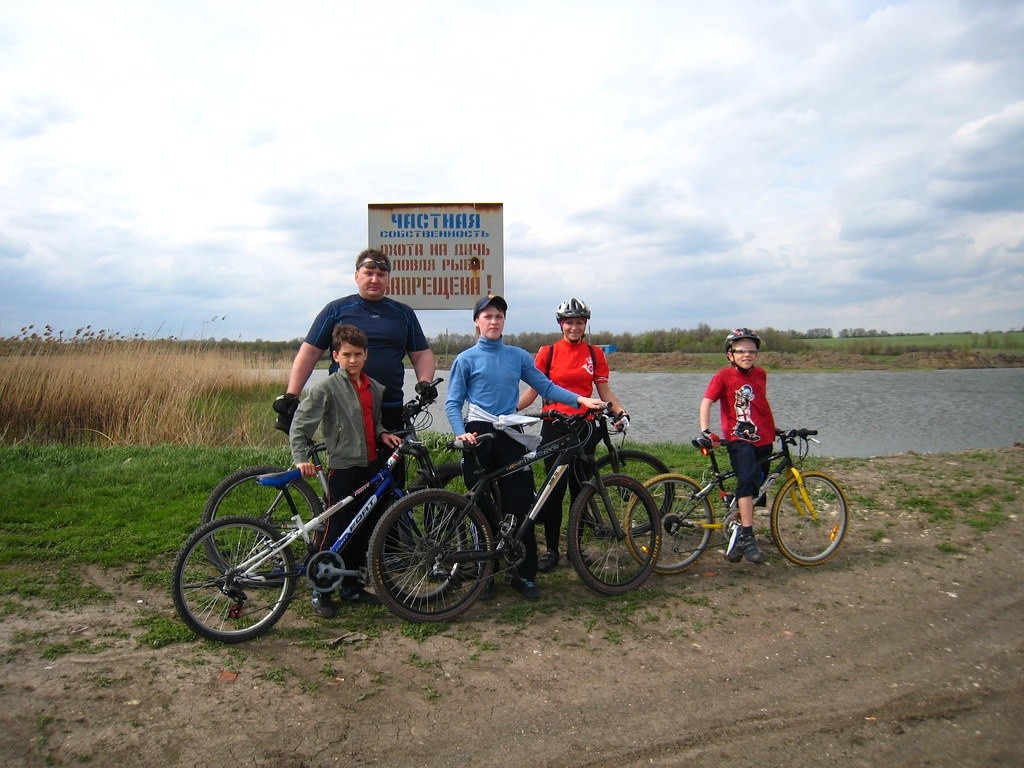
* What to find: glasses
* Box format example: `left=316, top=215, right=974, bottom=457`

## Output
left=357, top=261, right=389, bottom=271
left=730, top=348, right=757, bottom=354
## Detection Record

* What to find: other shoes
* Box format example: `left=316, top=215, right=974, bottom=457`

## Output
left=338, top=585, right=382, bottom=604
left=385, top=555, right=406, bottom=573
left=311, top=593, right=333, bottom=618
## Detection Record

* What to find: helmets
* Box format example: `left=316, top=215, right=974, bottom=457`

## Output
left=725, top=328, right=762, bottom=354
left=556, top=298, right=590, bottom=322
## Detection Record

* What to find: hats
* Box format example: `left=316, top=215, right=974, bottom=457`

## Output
left=473, top=294, right=507, bottom=321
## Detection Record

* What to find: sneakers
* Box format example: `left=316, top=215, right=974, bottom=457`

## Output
left=474, top=577, right=496, bottom=600
left=726, top=532, right=750, bottom=562
left=515, top=576, right=537, bottom=597
left=579, top=546, right=592, bottom=566
left=537, top=549, right=560, bottom=571
left=742, top=536, right=763, bottom=563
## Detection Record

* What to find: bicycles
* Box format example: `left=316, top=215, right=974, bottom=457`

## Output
left=167, top=377, right=676, bottom=645
left=623, top=428, right=849, bottom=576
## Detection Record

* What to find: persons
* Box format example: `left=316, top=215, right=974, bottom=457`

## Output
left=516, top=297, right=631, bottom=572
left=700, top=328, right=776, bottom=563
left=272, top=249, right=437, bottom=574
left=290, top=323, right=405, bottom=617
left=445, top=294, right=606, bottom=599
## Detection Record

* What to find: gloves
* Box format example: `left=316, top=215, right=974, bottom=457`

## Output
left=415, top=380, right=438, bottom=401
left=614, top=410, right=630, bottom=431
left=273, top=393, right=300, bottom=417
